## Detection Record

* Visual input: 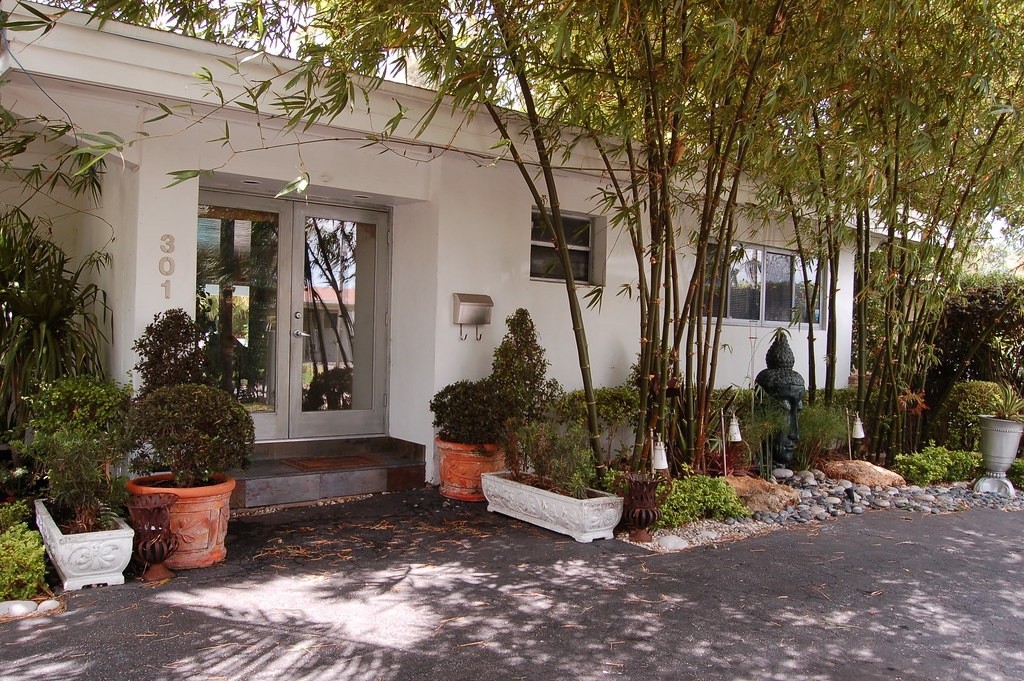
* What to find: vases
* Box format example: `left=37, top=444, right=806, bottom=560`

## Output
left=122, top=491, right=181, bottom=582
left=613, top=473, right=672, bottom=528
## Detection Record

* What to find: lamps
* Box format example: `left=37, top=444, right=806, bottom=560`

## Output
left=845, top=407, right=865, bottom=460
left=720, top=407, right=742, bottom=476
left=650, top=428, right=668, bottom=478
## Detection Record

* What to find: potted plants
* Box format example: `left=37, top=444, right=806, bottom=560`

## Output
left=428, top=377, right=526, bottom=502
left=121, top=383, right=257, bottom=570
left=973, top=378, right=1024, bottom=498
left=480, top=413, right=624, bottom=543
left=34, top=460, right=136, bottom=591
